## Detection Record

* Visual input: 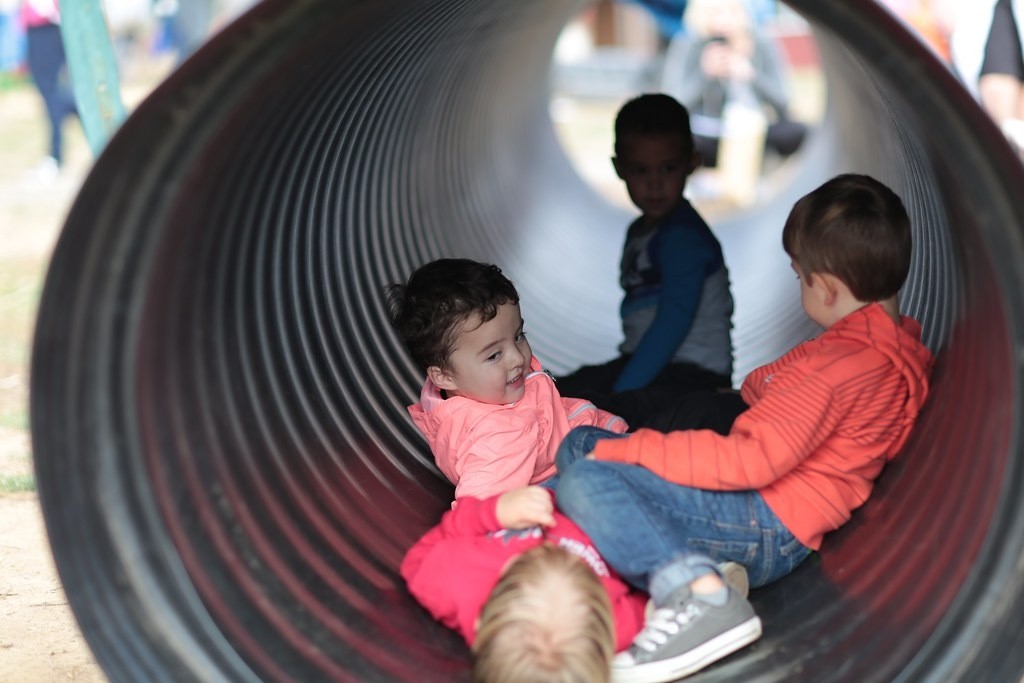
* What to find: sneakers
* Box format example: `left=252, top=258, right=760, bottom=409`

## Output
left=605, top=560, right=763, bottom=683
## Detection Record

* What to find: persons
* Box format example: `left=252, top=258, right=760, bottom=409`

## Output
left=679, top=1, right=796, bottom=204
left=380, top=257, right=632, bottom=512
left=544, top=91, right=737, bottom=433
left=1, top=0, right=189, bottom=179
left=551, top=173, right=936, bottom=681
left=398, top=472, right=653, bottom=682
